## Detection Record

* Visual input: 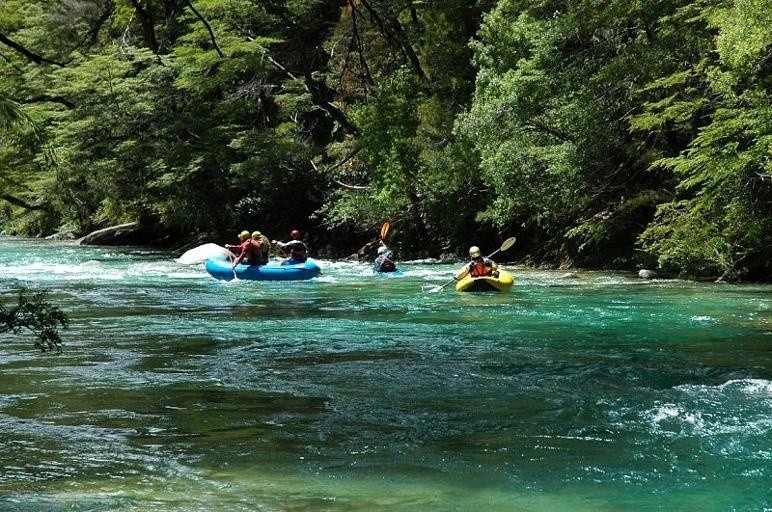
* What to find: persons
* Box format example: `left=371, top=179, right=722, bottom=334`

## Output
left=225, top=230, right=270, bottom=269
left=281, top=243, right=306, bottom=266
left=272, top=230, right=309, bottom=262
left=454, top=245, right=500, bottom=280
left=374, top=234, right=392, bottom=273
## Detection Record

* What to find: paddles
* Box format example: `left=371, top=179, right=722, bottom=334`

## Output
left=373, top=223, right=389, bottom=274
left=429, top=237, right=516, bottom=293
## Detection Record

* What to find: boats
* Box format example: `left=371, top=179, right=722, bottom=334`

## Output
left=377, top=256, right=396, bottom=272
left=456, top=268, right=514, bottom=292
left=205, top=255, right=320, bottom=281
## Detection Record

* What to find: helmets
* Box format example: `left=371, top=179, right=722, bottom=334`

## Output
left=469, top=246, right=481, bottom=258
left=291, top=230, right=301, bottom=239
left=252, top=231, right=262, bottom=238
left=241, top=230, right=250, bottom=239
